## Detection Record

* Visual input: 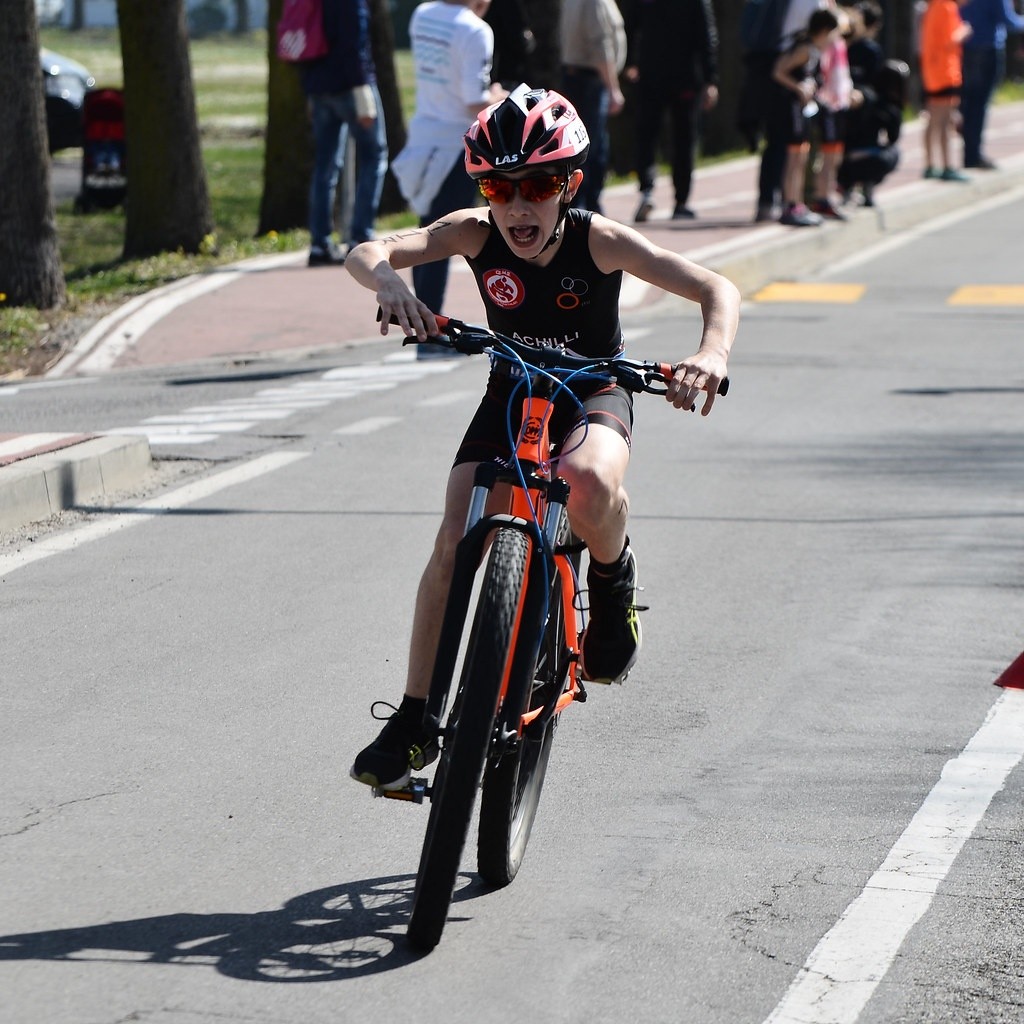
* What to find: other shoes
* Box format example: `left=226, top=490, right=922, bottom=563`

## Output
left=307, top=240, right=380, bottom=266
left=632, top=198, right=698, bottom=223
left=754, top=153, right=1002, bottom=228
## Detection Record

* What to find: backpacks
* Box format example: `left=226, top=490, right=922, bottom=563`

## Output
left=274, top=1, right=331, bottom=64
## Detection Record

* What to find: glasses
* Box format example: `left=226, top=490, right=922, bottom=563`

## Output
left=477, top=170, right=573, bottom=204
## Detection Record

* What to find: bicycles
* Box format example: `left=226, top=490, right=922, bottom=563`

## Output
left=369, top=305, right=733, bottom=950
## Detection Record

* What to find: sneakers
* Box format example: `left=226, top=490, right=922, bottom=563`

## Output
left=578, top=546, right=642, bottom=683
left=349, top=711, right=440, bottom=791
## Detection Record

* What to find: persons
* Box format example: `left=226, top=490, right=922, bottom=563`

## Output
left=558, top=0, right=629, bottom=220
left=960, top=0, right=1024, bottom=171
left=733, top=1, right=902, bottom=228
left=624, top=0, right=722, bottom=221
left=284, top=0, right=389, bottom=269
left=390, top=0, right=510, bottom=361
left=919, top=0, right=971, bottom=183
left=340, top=83, right=746, bottom=794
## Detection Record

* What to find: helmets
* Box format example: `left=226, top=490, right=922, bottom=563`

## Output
left=462, top=83, right=590, bottom=179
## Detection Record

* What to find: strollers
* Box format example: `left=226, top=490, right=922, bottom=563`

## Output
left=73, top=86, right=128, bottom=213
